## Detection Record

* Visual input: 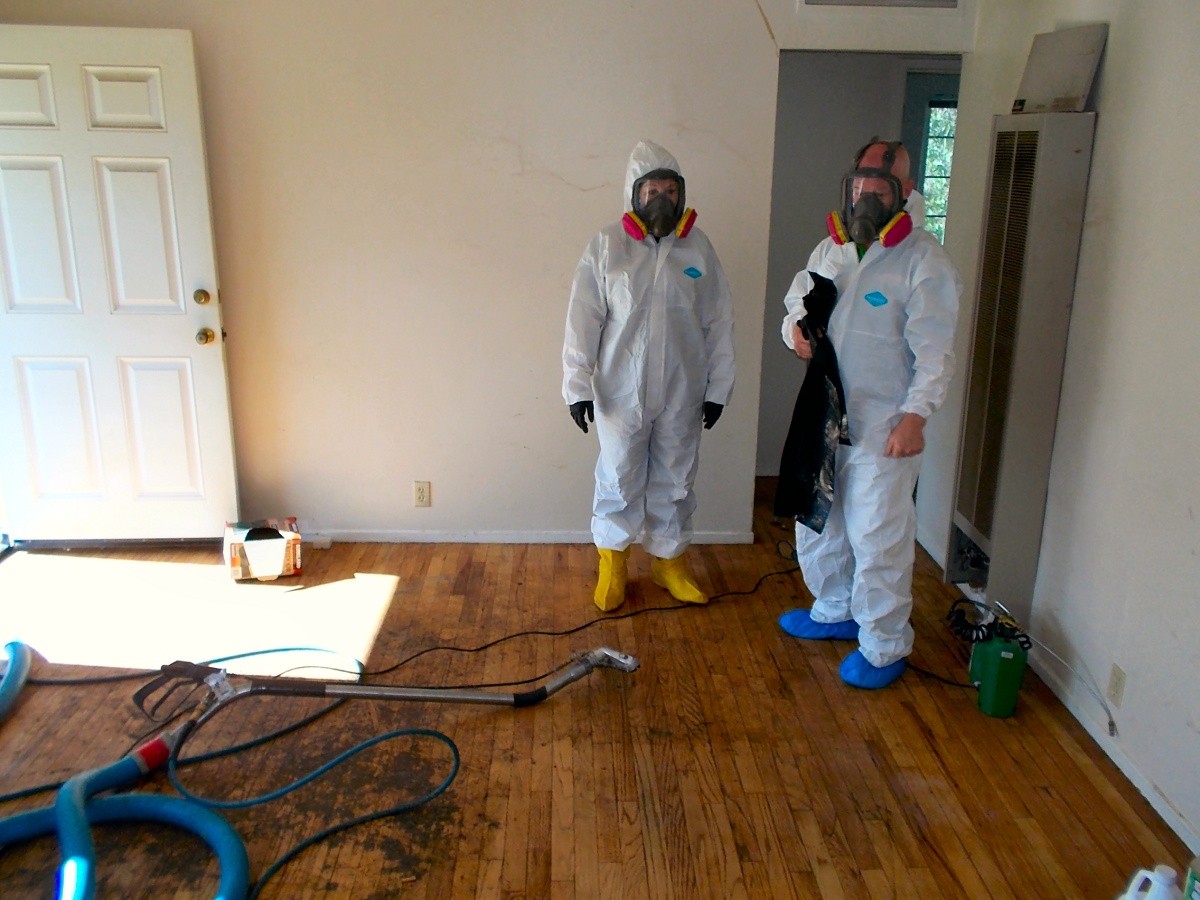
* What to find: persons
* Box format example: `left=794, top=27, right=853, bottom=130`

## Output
left=562, top=139, right=737, bottom=611
left=781, top=135, right=962, bottom=688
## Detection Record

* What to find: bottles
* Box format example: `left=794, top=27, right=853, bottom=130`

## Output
left=966, top=610, right=1025, bottom=718
left=1115, top=853, right=1200, bottom=900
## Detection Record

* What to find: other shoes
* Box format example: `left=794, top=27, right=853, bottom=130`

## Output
left=838, top=649, right=905, bottom=689
left=650, top=554, right=709, bottom=604
left=594, top=543, right=632, bottom=612
left=778, top=609, right=860, bottom=640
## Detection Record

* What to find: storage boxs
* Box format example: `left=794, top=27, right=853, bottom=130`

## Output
left=225, top=516, right=306, bottom=582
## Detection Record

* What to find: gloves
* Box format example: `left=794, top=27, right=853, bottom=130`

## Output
left=571, top=400, right=594, bottom=434
left=702, top=402, right=724, bottom=430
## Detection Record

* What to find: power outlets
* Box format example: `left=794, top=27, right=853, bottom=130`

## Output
left=416, top=478, right=431, bottom=510
